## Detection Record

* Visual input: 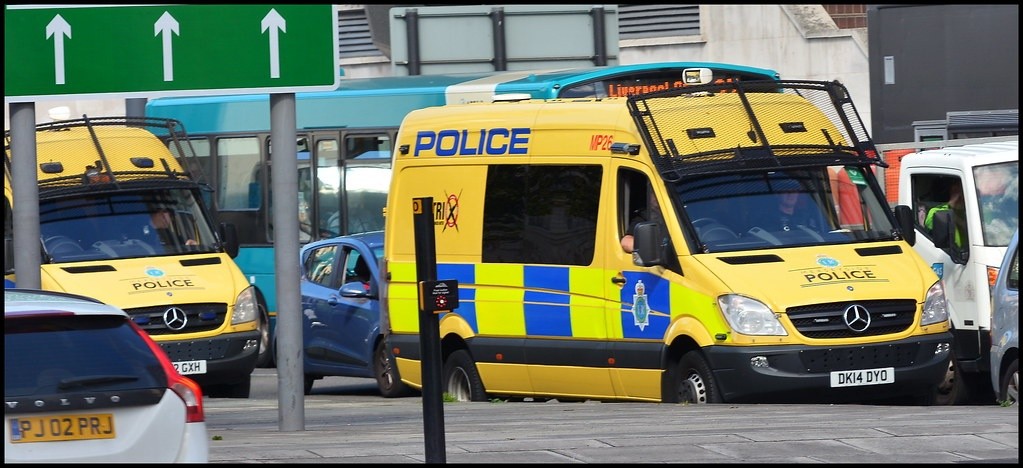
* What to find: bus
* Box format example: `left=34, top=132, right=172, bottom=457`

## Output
left=142, top=59, right=782, bottom=367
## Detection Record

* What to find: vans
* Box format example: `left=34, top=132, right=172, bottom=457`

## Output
left=382, top=77, right=953, bottom=408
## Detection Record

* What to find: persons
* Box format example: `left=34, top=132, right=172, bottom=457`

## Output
left=924, top=182, right=966, bottom=253
left=620, top=185, right=663, bottom=252
left=759, top=179, right=832, bottom=233
left=141, top=201, right=198, bottom=247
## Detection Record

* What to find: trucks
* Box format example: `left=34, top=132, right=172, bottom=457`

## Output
left=879, top=138, right=1022, bottom=406
left=3, top=114, right=272, bottom=400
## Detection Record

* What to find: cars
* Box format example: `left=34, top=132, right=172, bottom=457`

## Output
left=269, top=230, right=407, bottom=399
left=4, top=288, right=209, bottom=464
left=988, top=228, right=1019, bottom=407
